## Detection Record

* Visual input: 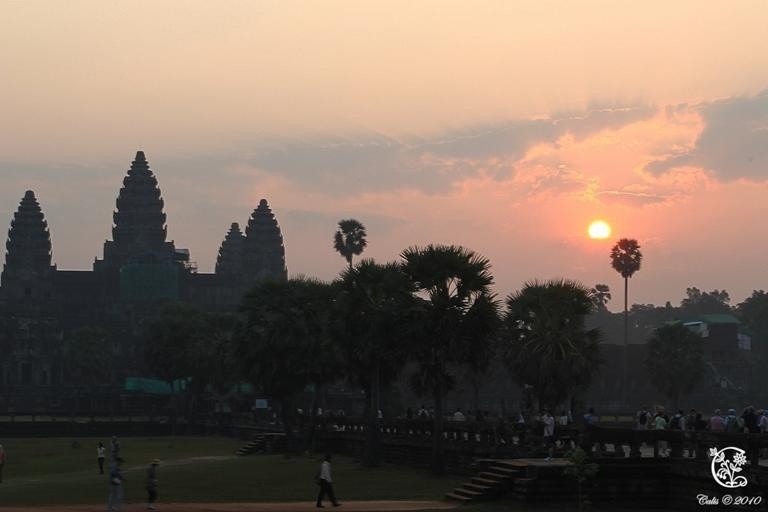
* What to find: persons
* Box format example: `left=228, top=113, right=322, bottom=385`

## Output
left=144, top=459, right=161, bottom=510
left=109, top=434, right=120, bottom=463
left=107, top=464, right=127, bottom=510
left=317, top=454, right=342, bottom=508
left=377, top=406, right=768, bottom=462
left=96, top=440, right=107, bottom=474
left=0, top=442, right=7, bottom=481
